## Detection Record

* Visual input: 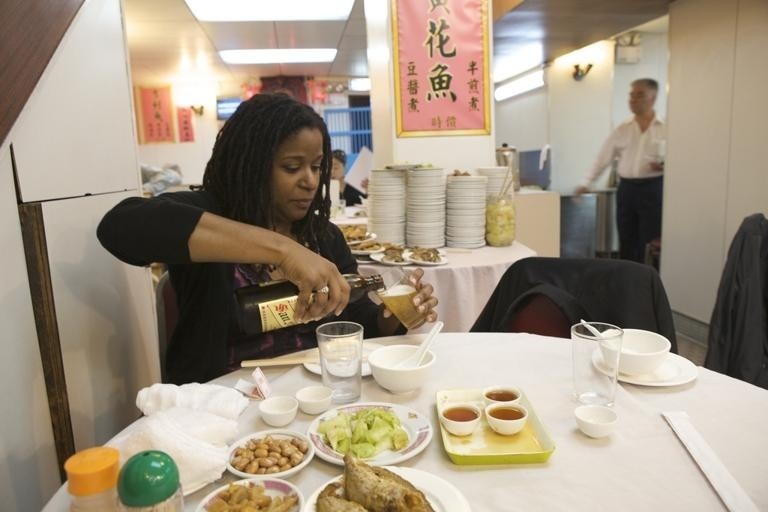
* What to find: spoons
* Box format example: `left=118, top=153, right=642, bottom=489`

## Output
left=393, top=321, right=444, bottom=368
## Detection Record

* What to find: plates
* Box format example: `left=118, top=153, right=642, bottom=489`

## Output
left=355, top=258, right=377, bottom=264
left=347, top=233, right=377, bottom=245
left=404, top=248, right=446, bottom=256
left=383, top=163, right=421, bottom=169
left=227, top=430, right=315, bottom=477
left=179, top=443, right=227, bottom=496
left=402, top=253, right=449, bottom=265
left=350, top=246, right=386, bottom=255
left=476, top=166, right=514, bottom=201
left=305, top=401, right=434, bottom=466
left=591, top=346, right=699, bottom=387
left=448, top=175, right=488, bottom=248
left=196, top=477, right=305, bottom=512
left=407, top=168, right=447, bottom=249
left=370, top=252, right=412, bottom=265
left=305, top=466, right=473, bottom=512
left=301, top=344, right=373, bottom=378
left=369, top=169, right=406, bottom=246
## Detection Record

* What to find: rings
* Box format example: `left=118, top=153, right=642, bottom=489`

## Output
left=318, top=286, right=329, bottom=294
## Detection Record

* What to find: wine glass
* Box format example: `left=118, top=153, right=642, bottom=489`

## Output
left=333, top=191, right=346, bottom=221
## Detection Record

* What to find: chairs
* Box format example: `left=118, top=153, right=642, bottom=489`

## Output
left=470, top=257, right=679, bottom=355
left=704, top=213, right=768, bottom=395
left=156, top=264, right=196, bottom=385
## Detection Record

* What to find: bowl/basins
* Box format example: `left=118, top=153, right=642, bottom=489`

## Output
left=599, top=329, right=672, bottom=375
left=574, top=405, right=619, bottom=439
left=258, top=395, right=298, bottom=427
left=482, top=386, right=522, bottom=405
left=485, top=402, right=529, bottom=437
left=438, top=401, right=481, bottom=436
left=296, top=386, right=333, bottom=415
left=368, top=345, right=436, bottom=395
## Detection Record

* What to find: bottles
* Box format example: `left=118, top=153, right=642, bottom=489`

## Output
left=118, top=449, right=187, bottom=512
left=487, top=191, right=516, bottom=248
left=232, top=274, right=384, bottom=343
left=64, top=447, right=120, bottom=511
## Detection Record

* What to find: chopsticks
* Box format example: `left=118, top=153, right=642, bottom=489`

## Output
left=242, top=356, right=322, bottom=369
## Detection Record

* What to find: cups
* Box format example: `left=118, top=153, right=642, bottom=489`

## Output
left=315, top=321, right=364, bottom=404
left=375, top=267, right=432, bottom=331
left=570, top=322, right=624, bottom=408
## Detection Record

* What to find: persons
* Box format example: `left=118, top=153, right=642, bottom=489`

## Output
left=331, top=150, right=369, bottom=207
left=572, top=78, right=666, bottom=273
left=95, top=92, right=438, bottom=385
